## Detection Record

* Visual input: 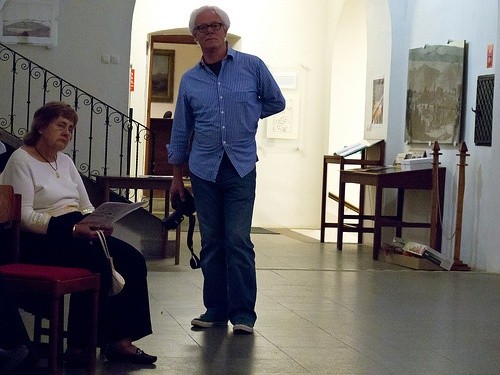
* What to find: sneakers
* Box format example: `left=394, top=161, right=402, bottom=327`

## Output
left=191, top=310, right=228, bottom=326
left=233, top=320, right=253, bottom=332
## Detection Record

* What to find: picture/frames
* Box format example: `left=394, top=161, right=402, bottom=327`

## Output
left=152, top=49, right=176, bottom=103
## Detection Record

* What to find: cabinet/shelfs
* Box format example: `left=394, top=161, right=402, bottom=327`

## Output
left=149, top=118, right=189, bottom=177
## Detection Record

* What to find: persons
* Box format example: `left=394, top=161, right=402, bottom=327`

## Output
left=166, top=7, right=285, bottom=332
left=0, top=101, right=157, bottom=375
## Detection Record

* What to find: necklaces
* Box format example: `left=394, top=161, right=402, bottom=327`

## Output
left=35, top=146, right=60, bottom=179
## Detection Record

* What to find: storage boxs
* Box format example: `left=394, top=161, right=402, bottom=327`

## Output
left=401, top=158, right=433, bottom=171
left=384, top=239, right=454, bottom=270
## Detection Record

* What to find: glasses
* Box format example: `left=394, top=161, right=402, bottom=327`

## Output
left=193, top=23, right=225, bottom=33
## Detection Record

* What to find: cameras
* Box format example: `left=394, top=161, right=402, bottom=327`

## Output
left=161, top=188, right=196, bottom=230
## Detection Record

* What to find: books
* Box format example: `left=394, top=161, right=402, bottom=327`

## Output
left=333, top=143, right=367, bottom=157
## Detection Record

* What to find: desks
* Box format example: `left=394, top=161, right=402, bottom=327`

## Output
left=338, top=165, right=446, bottom=261
left=321, top=145, right=384, bottom=249
left=89, top=172, right=194, bottom=266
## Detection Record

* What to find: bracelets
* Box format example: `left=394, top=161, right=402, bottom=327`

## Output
left=73, top=226, right=76, bottom=238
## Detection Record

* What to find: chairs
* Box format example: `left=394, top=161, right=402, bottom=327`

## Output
left=0, top=184, right=104, bottom=375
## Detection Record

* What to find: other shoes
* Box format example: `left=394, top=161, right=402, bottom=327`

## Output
left=99, top=345, right=158, bottom=364
